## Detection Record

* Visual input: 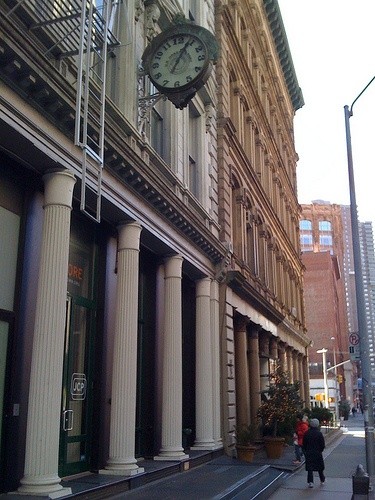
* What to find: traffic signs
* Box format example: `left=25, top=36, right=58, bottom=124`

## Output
left=349, top=332, right=361, bottom=361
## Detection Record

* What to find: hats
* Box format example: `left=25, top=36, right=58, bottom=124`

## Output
left=309, top=419, right=320, bottom=428
left=296, top=413, right=303, bottom=421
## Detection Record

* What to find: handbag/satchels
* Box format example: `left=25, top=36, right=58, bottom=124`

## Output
left=293, top=432, right=299, bottom=445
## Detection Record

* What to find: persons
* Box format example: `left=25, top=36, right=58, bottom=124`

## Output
left=293, top=416, right=309, bottom=464
left=301, top=418, right=325, bottom=488
left=351, top=405, right=363, bottom=417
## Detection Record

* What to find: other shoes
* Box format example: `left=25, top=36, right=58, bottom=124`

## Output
left=308, top=481, right=314, bottom=488
left=320, top=480, right=327, bottom=486
left=293, top=461, right=301, bottom=464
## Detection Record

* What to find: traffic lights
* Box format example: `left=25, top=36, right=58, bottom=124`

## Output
left=338, top=375, right=343, bottom=384
left=315, top=393, right=325, bottom=401
left=329, top=397, right=335, bottom=403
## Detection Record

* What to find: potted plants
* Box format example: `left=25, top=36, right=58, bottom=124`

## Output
left=231, top=421, right=256, bottom=461
left=259, top=369, right=291, bottom=458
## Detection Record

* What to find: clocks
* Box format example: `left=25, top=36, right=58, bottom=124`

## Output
left=139, top=12, right=220, bottom=108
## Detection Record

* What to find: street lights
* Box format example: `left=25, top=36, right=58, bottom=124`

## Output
left=330, top=336, right=339, bottom=421
